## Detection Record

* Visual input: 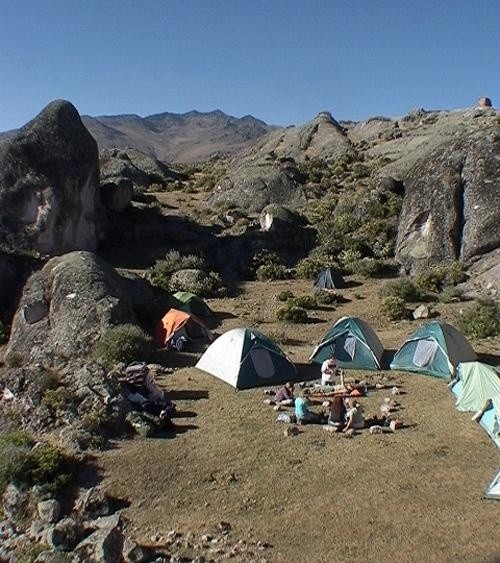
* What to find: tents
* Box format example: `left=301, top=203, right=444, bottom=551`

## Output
left=194, top=328, right=297, bottom=391
left=481, top=470, right=500, bottom=501
left=389, top=321, right=479, bottom=381
left=312, top=267, right=347, bottom=289
left=447, top=362, right=500, bottom=451
left=308, top=317, right=384, bottom=372
left=156, top=291, right=217, bottom=354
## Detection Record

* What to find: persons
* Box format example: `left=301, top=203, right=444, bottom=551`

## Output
left=272, top=352, right=366, bottom=429
